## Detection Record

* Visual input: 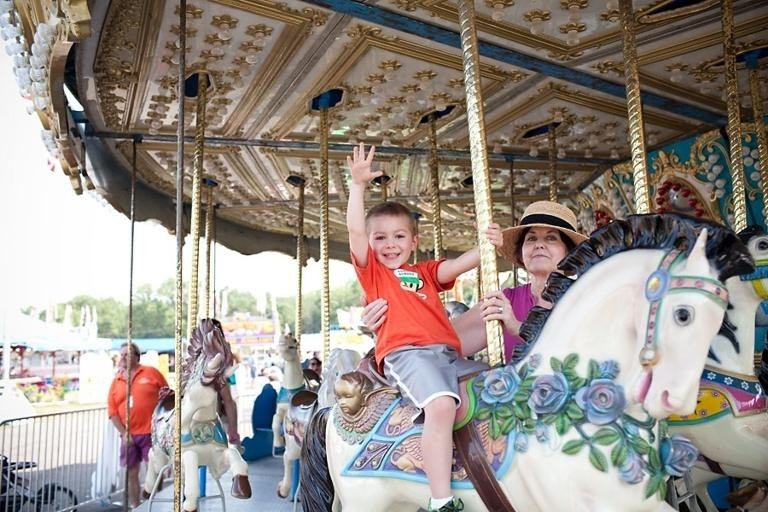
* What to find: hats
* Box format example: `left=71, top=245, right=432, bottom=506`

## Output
left=498, top=200, right=590, bottom=267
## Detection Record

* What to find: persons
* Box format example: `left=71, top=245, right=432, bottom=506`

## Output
left=345, top=143, right=503, bottom=512
left=108, top=343, right=169, bottom=508
left=358, top=201, right=588, bottom=364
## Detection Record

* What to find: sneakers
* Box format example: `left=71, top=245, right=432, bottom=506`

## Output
left=428, top=496, right=464, bottom=512
